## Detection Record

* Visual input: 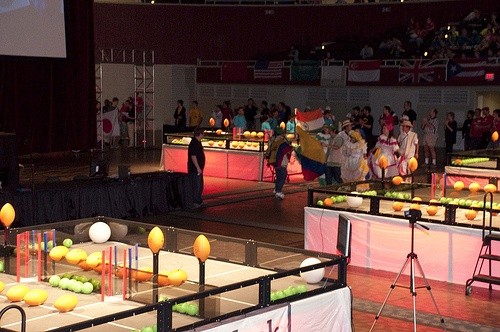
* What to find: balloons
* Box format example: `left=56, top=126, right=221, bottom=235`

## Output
left=347, top=191, right=363, bottom=208
left=299, top=257, right=325, bottom=284
left=88, top=221, right=112, bottom=243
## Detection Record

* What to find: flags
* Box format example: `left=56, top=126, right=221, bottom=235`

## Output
left=295, top=123, right=327, bottom=182
left=219, top=58, right=487, bottom=85
left=294, top=107, right=325, bottom=134
left=96, top=108, right=121, bottom=142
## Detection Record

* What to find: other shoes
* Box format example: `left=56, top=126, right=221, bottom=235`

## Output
left=199, top=202, right=208, bottom=207
left=275, top=192, right=284, bottom=200
left=273, top=189, right=282, bottom=194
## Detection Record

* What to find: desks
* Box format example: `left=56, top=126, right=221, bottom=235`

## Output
left=0, top=172, right=193, bottom=228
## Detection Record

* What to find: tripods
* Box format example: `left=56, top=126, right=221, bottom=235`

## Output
left=368, top=225, right=444, bottom=332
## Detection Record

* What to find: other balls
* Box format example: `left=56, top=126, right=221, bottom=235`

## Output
left=0, top=129, right=500, bottom=332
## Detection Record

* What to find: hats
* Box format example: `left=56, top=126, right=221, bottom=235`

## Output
left=399, top=121, right=413, bottom=128
left=341, top=120, right=353, bottom=127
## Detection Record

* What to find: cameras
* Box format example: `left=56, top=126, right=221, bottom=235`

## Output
left=404, top=209, right=422, bottom=219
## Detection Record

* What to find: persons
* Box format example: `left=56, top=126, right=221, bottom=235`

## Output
left=174, top=98, right=500, bottom=199
left=142, top=0, right=500, bottom=63
left=188, top=127, right=208, bottom=209
left=95, top=97, right=144, bottom=150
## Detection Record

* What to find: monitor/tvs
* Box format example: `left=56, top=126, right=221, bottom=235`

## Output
left=89, top=159, right=109, bottom=178
